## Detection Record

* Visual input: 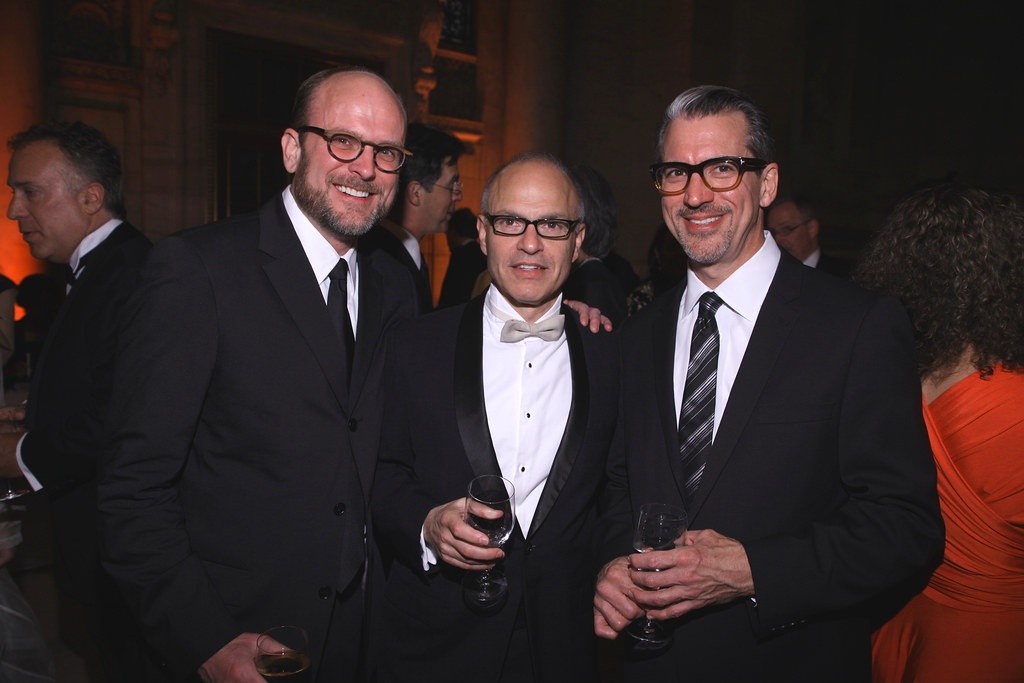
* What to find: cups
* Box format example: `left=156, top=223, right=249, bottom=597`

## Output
left=255, top=626, right=310, bottom=683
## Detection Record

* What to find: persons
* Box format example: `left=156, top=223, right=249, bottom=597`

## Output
left=0, top=122, right=1024, bottom=682
left=98, top=64, right=612, bottom=682
left=565, top=86, right=947, bottom=683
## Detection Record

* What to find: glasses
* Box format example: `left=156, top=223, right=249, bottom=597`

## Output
left=766, top=219, right=811, bottom=236
left=296, top=125, right=414, bottom=174
left=484, top=211, right=582, bottom=240
left=434, top=181, right=464, bottom=194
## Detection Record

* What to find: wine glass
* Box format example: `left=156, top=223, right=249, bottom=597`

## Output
left=624, top=502, right=688, bottom=641
left=0, top=375, right=30, bottom=502
left=464, top=475, right=515, bottom=609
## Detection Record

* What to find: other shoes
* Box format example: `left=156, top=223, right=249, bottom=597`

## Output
left=59, top=591, right=87, bottom=658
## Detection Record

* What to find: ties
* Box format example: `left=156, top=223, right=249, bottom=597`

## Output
left=326, top=258, right=356, bottom=396
left=420, top=252, right=433, bottom=307
left=677, top=292, right=722, bottom=496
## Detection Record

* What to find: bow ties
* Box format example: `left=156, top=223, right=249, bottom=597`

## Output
left=499, top=314, right=566, bottom=342
left=61, top=264, right=79, bottom=285
